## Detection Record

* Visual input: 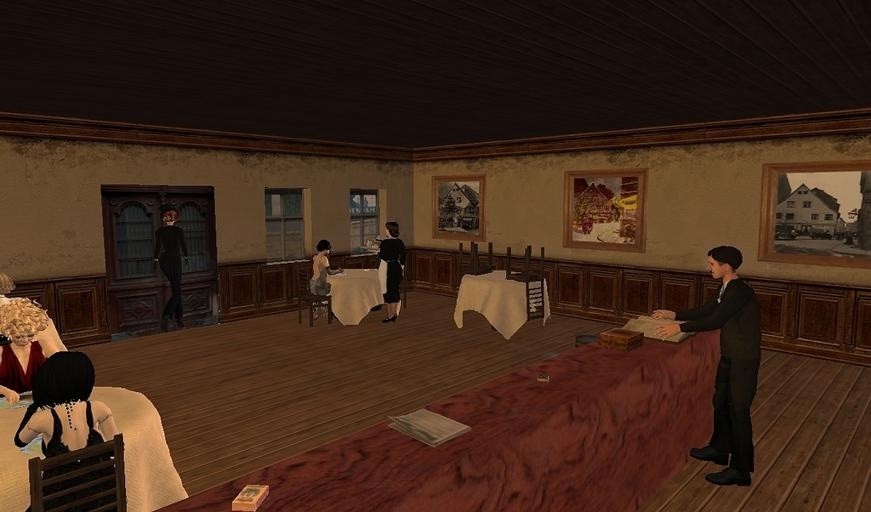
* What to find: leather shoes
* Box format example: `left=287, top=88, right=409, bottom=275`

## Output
left=692, top=440, right=752, bottom=487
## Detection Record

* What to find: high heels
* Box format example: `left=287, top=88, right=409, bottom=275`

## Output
left=384, top=313, right=396, bottom=323
